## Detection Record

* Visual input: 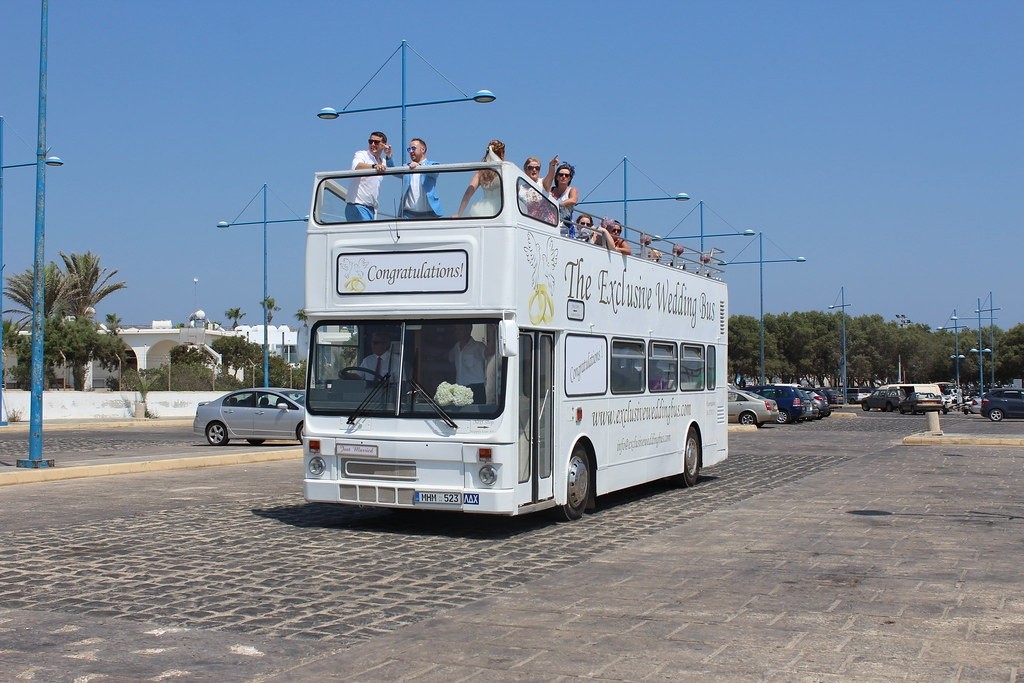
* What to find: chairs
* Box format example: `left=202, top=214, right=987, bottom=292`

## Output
left=260, top=398, right=273, bottom=407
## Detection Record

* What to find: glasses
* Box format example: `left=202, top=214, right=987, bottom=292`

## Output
left=612, top=229, right=621, bottom=233
left=528, top=166, right=540, bottom=170
left=557, top=173, right=570, bottom=177
left=580, top=222, right=591, bottom=226
left=407, top=147, right=422, bottom=152
left=368, top=139, right=382, bottom=144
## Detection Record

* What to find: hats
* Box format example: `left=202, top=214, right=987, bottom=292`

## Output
left=454, top=324, right=472, bottom=333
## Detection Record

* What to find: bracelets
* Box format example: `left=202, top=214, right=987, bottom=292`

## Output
left=371, top=163, right=377, bottom=168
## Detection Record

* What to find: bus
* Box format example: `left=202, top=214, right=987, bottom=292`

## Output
left=299, top=161, right=733, bottom=525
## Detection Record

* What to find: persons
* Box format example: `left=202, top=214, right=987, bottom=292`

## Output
left=518, top=153, right=560, bottom=225
left=549, top=161, right=578, bottom=238
left=594, top=220, right=631, bottom=255
left=379, top=138, right=444, bottom=218
left=638, top=359, right=668, bottom=390
left=574, top=214, right=615, bottom=249
left=741, top=379, right=746, bottom=388
left=648, top=247, right=662, bottom=262
left=437, top=324, right=495, bottom=404
left=344, top=132, right=387, bottom=222
left=338, top=330, right=407, bottom=403
left=450, top=138, right=521, bottom=218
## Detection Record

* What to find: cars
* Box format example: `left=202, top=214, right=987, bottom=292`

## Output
left=728, top=381, right=1024, bottom=429
left=192, top=386, right=306, bottom=446
left=728, top=389, right=780, bottom=428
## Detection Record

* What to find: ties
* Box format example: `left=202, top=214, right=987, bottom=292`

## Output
left=374, top=357, right=381, bottom=379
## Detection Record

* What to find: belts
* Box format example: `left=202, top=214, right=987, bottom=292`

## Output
left=404, top=210, right=434, bottom=216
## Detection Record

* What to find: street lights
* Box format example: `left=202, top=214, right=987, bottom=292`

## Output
left=216, top=182, right=310, bottom=388
left=0, top=115, right=65, bottom=428
left=974, top=290, right=1002, bottom=389
left=316, top=38, right=499, bottom=219
left=573, top=155, right=691, bottom=246
left=936, top=308, right=968, bottom=390
left=717, top=230, right=806, bottom=386
left=950, top=296, right=998, bottom=399
left=652, top=199, right=756, bottom=264
left=828, top=285, right=852, bottom=405
